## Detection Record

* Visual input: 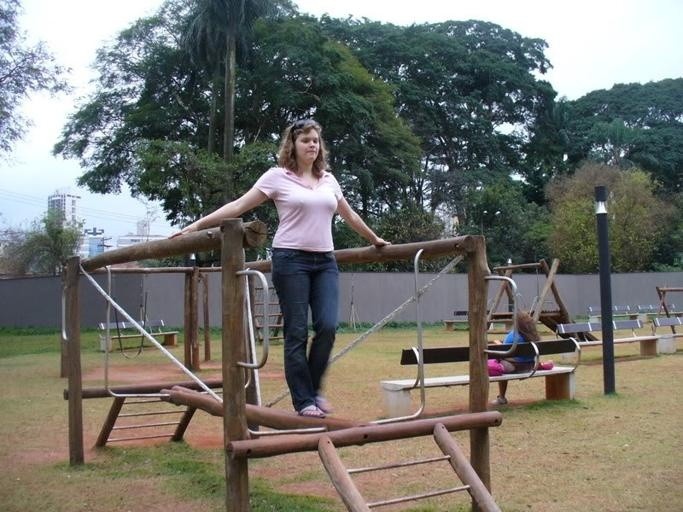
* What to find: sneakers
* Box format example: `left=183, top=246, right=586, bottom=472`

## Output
left=492, top=398, right=507, bottom=405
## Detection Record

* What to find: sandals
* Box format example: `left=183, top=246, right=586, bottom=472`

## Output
left=298, top=396, right=336, bottom=417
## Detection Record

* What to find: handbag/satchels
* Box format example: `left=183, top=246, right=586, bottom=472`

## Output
left=487, top=359, right=504, bottom=376
left=536, top=361, right=553, bottom=370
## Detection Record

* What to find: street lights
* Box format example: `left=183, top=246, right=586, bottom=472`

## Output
left=595, top=185, right=616, bottom=395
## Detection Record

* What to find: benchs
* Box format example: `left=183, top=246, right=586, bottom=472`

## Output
left=444, top=308, right=491, bottom=333
left=97, top=320, right=181, bottom=351
left=553, top=315, right=683, bottom=361
left=376, top=336, right=590, bottom=415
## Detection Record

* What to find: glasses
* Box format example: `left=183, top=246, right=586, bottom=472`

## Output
left=296, top=120, right=315, bottom=127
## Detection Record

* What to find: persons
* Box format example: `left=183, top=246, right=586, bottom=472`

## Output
left=168, top=119, right=392, bottom=418
left=489, top=310, right=541, bottom=406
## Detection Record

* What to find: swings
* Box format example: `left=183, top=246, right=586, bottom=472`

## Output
left=112, top=273, right=151, bottom=359
left=519, top=268, right=538, bottom=303
